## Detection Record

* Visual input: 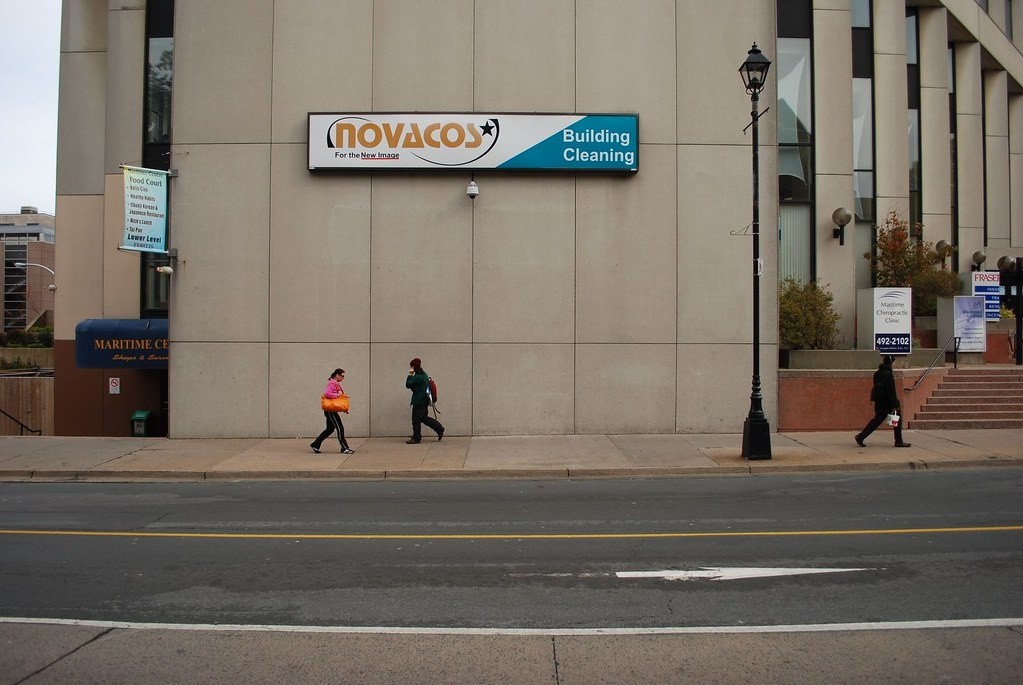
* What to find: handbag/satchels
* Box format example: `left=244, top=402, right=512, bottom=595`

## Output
left=886, top=410, right=900, bottom=427
left=429, top=377, right=437, bottom=403
left=321, top=384, right=350, bottom=412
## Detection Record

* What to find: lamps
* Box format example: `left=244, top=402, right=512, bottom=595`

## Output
left=894, top=227, right=909, bottom=240
left=831, top=208, right=851, bottom=247
left=935, top=240, right=951, bottom=267
left=972, top=251, right=986, bottom=272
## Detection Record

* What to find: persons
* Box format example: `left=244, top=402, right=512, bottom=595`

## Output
left=310, top=369, right=355, bottom=454
left=855, top=355, right=911, bottom=448
left=405, top=357, right=445, bottom=444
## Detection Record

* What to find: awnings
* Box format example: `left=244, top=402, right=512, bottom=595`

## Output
left=76, top=319, right=169, bottom=370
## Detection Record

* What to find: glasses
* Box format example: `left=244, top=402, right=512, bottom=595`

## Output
left=338, top=374, right=344, bottom=378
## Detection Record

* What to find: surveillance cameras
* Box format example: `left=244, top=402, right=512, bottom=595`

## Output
left=466, top=181, right=479, bottom=199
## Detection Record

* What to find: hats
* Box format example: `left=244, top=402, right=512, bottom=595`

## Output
left=883, top=355, right=895, bottom=365
left=410, top=358, right=421, bottom=368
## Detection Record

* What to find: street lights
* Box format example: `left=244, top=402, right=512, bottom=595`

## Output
left=737, top=39, right=773, bottom=460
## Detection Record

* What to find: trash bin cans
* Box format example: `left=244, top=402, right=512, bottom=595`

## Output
left=129, top=409, right=153, bottom=437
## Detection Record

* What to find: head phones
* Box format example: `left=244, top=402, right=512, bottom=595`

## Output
left=889, top=355, right=892, bottom=365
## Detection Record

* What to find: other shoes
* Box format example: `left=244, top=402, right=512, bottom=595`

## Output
left=310, top=446, right=321, bottom=453
left=855, top=435, right=866, bottom=447
left=406, top=439, right=420, bottom=444
left=341, top=448, right=354, bottom=454
left=895, top=441, right=911, bottom=447
left=438, top=427, right=444, bottom=441
